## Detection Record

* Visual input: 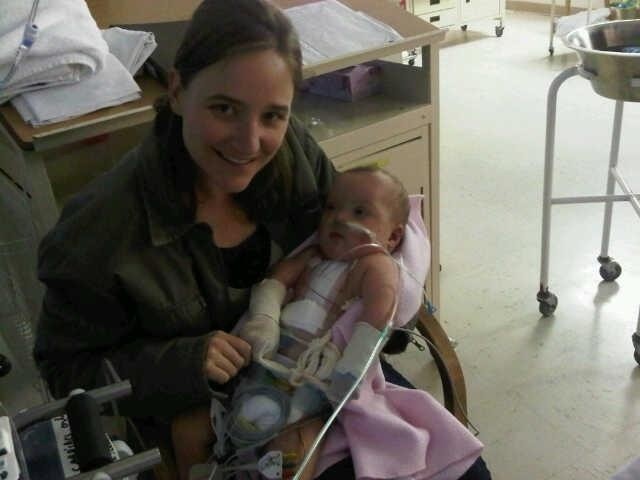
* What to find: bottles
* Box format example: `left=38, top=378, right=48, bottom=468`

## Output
left=64, top=387, right=112, bottom=472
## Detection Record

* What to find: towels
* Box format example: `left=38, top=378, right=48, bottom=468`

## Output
left=1, top=0, right=110, bottom=101
left=98, top=25, right=157, bottom=76
left=11, top=49, right=143, bottom=131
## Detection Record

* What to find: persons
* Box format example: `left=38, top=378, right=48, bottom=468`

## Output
left=32, top=0, right=492, bottom=480
left=219, top=165, right=411, bottom=452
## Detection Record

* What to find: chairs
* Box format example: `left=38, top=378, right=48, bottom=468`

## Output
left=415, top=306, right=468, bottom=426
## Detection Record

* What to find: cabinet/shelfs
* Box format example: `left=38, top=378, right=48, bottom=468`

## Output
left=1, top=0, right=444, bottom=328
left=408, top=1, right=508, bottom=38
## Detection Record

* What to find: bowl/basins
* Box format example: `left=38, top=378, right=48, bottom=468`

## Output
left=561, top=17, right=640, bottom=102
